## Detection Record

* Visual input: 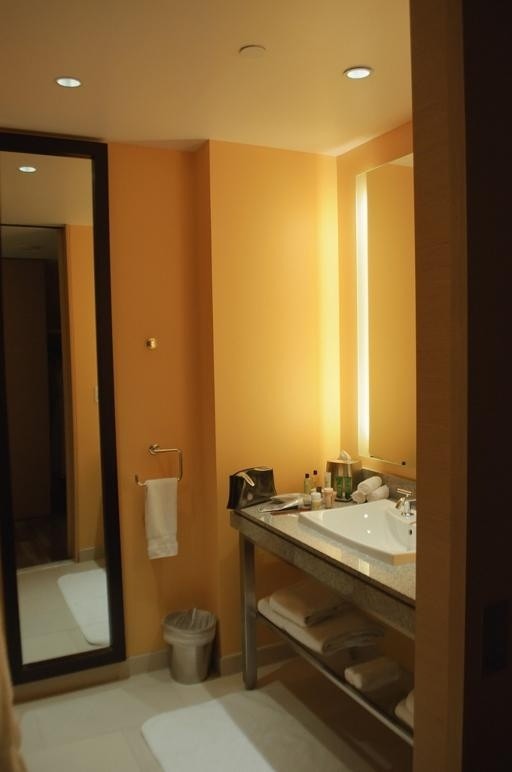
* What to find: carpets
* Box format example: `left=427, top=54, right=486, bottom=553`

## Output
left=56, top=567, right=110, bottom=646
left=140, top=678, right=412, bottom=771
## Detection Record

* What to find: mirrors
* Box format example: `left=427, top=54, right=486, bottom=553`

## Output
left=355, top=153, right=416, bottom=469
left=0, top=133, right=126, bottom=687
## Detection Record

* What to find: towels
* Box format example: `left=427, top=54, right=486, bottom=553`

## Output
left=351, top=476, right=389, bottom=504
left=257, top=576, right=386, bottom=655
left=144, top=478, right=178, bottom=559
left=343, top=656, right=401, bottom=692
left=393, top=689, right=414, bottom=730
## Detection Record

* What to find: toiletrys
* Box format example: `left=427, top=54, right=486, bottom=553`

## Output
left=257, top=468, right=337, bottom=515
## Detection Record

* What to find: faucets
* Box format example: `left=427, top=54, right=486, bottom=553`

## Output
left=395, top=486, right=416, bottom=515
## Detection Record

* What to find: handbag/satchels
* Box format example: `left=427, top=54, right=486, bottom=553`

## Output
left=326, top=458, right=363, bottom=503
left=226, top=467, right=276, bottom=510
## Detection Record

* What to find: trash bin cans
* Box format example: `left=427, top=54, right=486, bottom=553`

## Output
left=162, top=607, right=217, bottom=684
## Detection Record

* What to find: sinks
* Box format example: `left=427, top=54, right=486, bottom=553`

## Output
left=299, top=498, right=417, bottom=565
